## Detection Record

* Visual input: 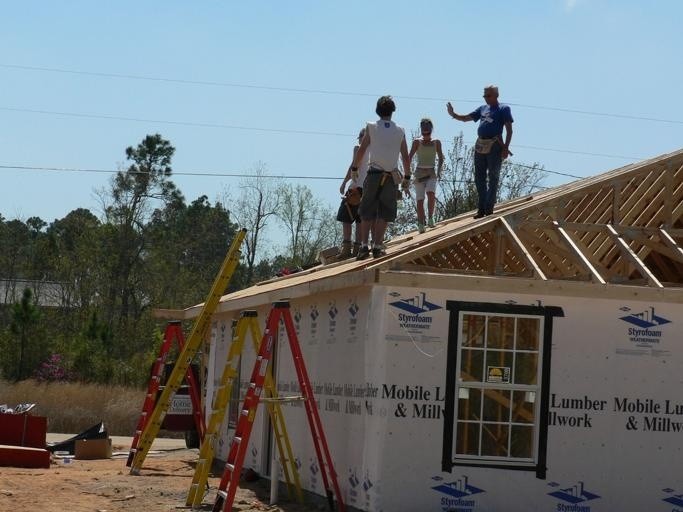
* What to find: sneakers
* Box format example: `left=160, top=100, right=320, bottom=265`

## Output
left=371, top=248, right=380, bottom=258
left=428, top=219, right=436, bottom=228
left=356, top=246, right=368, bottom=260
left=418, top=224, right=425, bottom=234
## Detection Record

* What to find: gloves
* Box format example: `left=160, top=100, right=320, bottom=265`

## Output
left=401, top=176, right=410, bottom=193
left=350, top=167, right=358, bottom=183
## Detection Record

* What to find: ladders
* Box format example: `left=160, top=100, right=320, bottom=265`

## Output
left=126, top=320, right=207, bottom=467
left=128, top=228, right=248, bottom=476
left=211, top=300, right=346, bottom=512
left=185, top=310, right=307, bottom=510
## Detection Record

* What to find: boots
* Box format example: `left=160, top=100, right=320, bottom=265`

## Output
left=335, top=240, right=351, bottom=259
left=352, top=243, right=359, bottom=257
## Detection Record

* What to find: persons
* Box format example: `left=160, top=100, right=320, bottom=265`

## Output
left=348, top=95, right=411, bottom=260
left=407, top=117, right=444, bottom=235
left=333, top=127, right=371, bottom=260
left=444, top=83, right=514, bottom=220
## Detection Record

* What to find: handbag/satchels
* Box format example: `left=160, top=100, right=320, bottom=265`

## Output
left=474, top=138, right=493, bottom=154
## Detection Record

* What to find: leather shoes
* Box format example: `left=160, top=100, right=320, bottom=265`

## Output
left=473, top=210, right=484, bottom=219
left=484, top=208, right=493, bottom=216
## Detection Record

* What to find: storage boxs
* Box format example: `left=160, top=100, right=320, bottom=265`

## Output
left=75, top=439, right=112, bottom=460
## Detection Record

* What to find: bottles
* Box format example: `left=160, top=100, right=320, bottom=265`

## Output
left=60, top=458, right=73, bottom=464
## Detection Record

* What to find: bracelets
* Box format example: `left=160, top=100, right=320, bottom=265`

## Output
left=350, top=166, right=358, bottom=172
left=452, top=113, right=457, bottom=119
left=403, top=174, right=410, bottom=181
